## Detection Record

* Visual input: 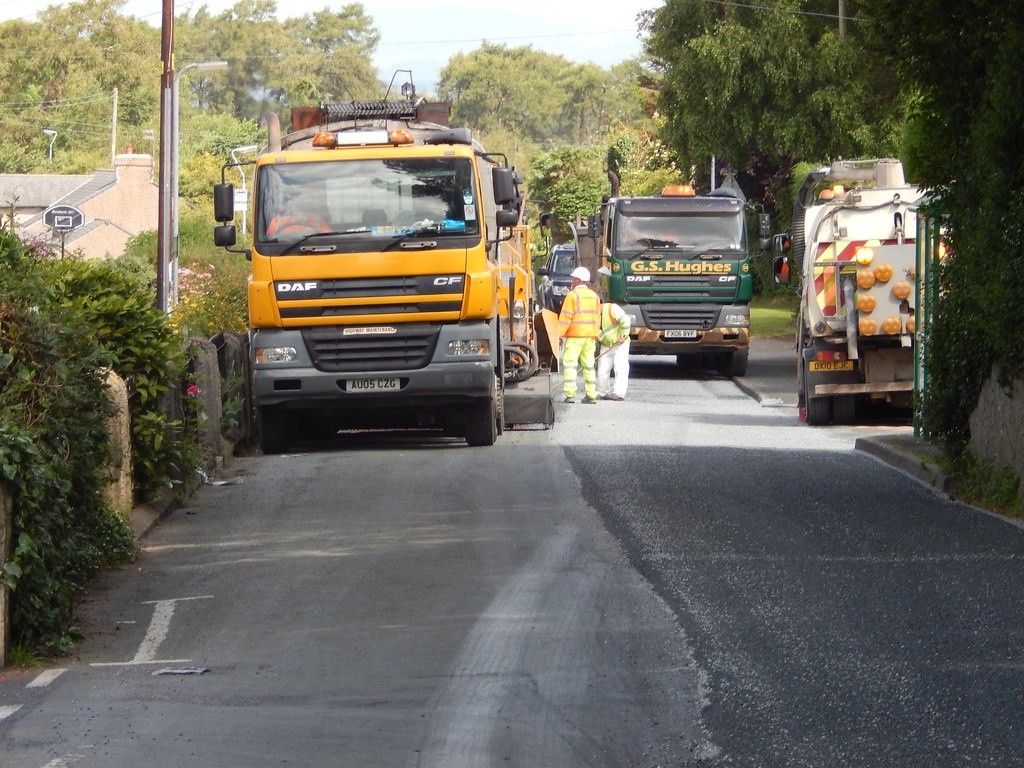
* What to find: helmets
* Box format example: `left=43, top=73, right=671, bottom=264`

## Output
left=569, top=267, right=590, bottom=282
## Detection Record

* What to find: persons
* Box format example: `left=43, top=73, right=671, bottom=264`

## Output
left=267, top=184, right=331, bottom=237
left=557, top=267, right=601, bottom=404
left=596, top=299, right=631, bottom=402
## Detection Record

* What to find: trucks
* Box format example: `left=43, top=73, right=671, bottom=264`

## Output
left=213, top=70, right=541, bottom=446
left=576, top=184, right=770, bottom=377
left=772, top=158, right=951, bottom=426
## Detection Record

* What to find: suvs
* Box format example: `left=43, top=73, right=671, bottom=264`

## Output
left=537, top=243, right=575, bottom=313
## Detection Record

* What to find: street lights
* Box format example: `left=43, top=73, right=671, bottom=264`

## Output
left=43, top=129, right=57, bottom=163
left=171, top=61, right=228, bottom=306
left=230, top=145, right=257, bottom=235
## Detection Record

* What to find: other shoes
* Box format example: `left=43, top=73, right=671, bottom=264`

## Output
left=581, top=396, right=597, bottom=404
left=596, top=394, right=605, bottom=400
left=564, top=398, right=575, bottom=403
left=605, top=394, right=624, bottom=401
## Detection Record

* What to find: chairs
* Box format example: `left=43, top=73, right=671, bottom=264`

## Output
left=362, top=209, right=388, bottom=226
